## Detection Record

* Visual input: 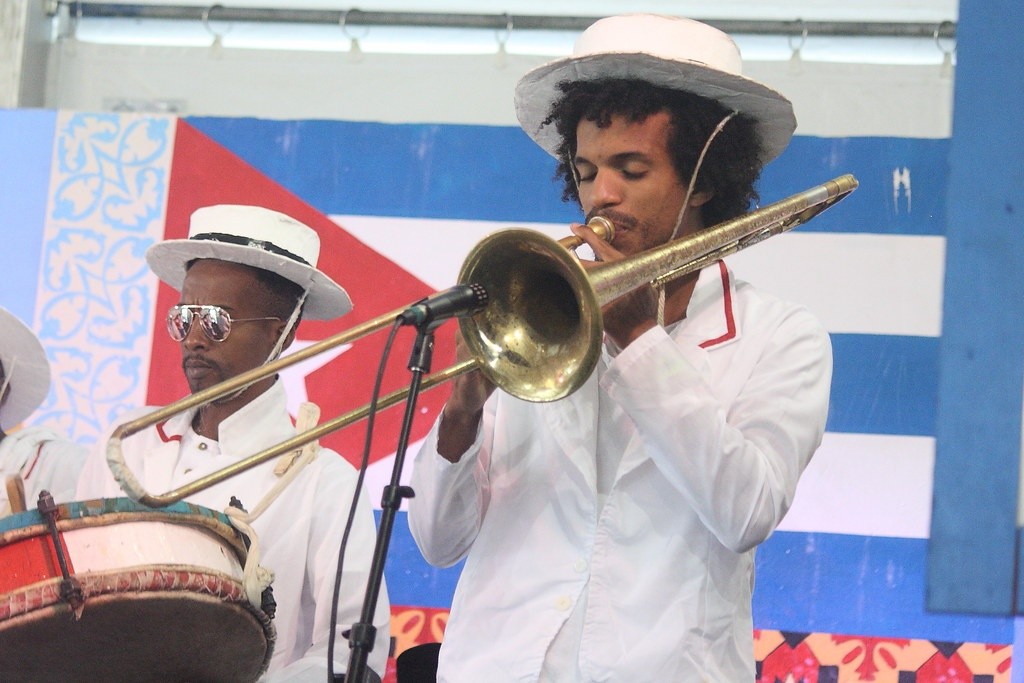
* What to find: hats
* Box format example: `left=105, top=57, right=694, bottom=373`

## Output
left=146, top=203, right=353, bottom=321
left=514, top=14, right=799, bottom=166
left=1, top=307, right=50, bottom=432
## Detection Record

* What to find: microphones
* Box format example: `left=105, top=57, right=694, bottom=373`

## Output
left=396, top=284, right=487, bottom=329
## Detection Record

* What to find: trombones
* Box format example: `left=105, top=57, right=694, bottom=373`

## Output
left=106, top=172, right=860, bottom=510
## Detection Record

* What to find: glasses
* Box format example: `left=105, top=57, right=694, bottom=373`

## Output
left=166, top=305, right=279, bottom=342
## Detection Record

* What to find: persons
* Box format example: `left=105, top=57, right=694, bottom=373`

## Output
left=74, top=204, right=391, bottom=683
left=0, top=307, right=90, bottom=520
left=407, top=11, right=833, bottom=683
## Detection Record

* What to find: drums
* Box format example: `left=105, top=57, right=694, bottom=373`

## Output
left=0, top=494, right=277, bottom=683
